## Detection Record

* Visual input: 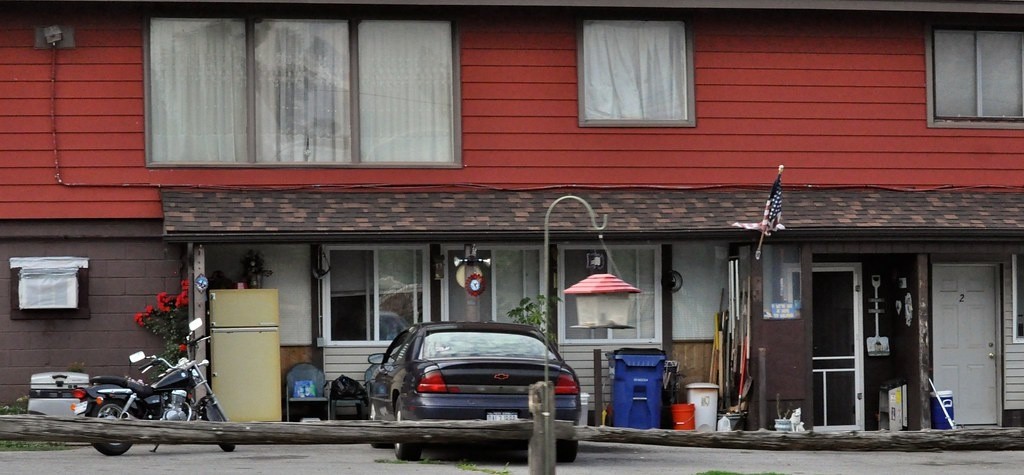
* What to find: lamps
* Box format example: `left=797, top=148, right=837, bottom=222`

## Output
left=454, top=256, right=492, bottom=267
left=313, top=252, right=333, bottom=279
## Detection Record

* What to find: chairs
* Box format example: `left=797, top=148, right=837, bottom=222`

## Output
left=287, top=363, right=331, bottom=422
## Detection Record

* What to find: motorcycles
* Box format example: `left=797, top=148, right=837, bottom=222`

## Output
left=70, top=317, right=235, bottom=456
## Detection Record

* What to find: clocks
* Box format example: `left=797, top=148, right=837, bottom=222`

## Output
left=470, top=280, right=481, bottom=291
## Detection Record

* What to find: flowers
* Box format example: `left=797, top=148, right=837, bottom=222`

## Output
left=134, top=278, right=194, bottom=351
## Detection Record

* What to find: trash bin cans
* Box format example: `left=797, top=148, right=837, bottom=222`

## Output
left=605, top=347, right=667, bottom=429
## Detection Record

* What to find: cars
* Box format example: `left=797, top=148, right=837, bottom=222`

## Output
left=364, top=321, right=581, bottom=463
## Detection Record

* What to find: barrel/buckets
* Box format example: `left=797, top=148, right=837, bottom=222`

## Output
left=930, top=391, right=954, bottom=429
left=718, top=413, right=743, bottom=431
left=670, top=403, right=695, bottom=431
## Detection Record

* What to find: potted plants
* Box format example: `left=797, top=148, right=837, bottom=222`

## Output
left=238, top=246, right=272, bottom=288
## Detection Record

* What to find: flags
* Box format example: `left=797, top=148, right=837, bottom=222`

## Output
left=732, top=176, right=782, bottom=235
